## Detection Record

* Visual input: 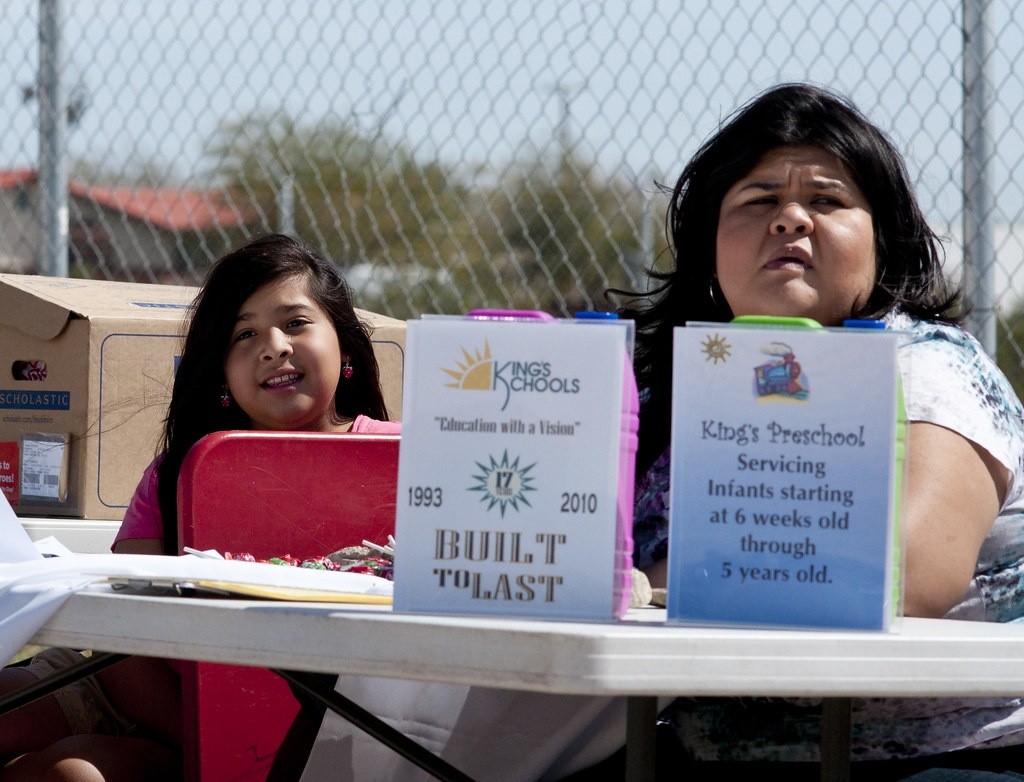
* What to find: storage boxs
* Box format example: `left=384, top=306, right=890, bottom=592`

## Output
left=0, top=273, right=406, bottom=521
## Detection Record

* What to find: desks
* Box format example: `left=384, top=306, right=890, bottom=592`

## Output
left=0, top=580, right=1024, bottom=782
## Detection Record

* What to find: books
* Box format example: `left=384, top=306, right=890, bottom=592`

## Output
left=666, top=327, right=896, bottom=637
left=393, top=318, right=625, bottom=628
left=200, top=580, right=395, bottom=605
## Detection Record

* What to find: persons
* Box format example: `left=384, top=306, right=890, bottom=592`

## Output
left=610, top=85, right=1023, bottom=781
left=0, top=233, right=402, bottom=781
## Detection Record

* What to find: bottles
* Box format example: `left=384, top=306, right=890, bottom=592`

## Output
left=569, top=307, right=640, bottom=618
left=840, top=316, right=912, bottom=624
